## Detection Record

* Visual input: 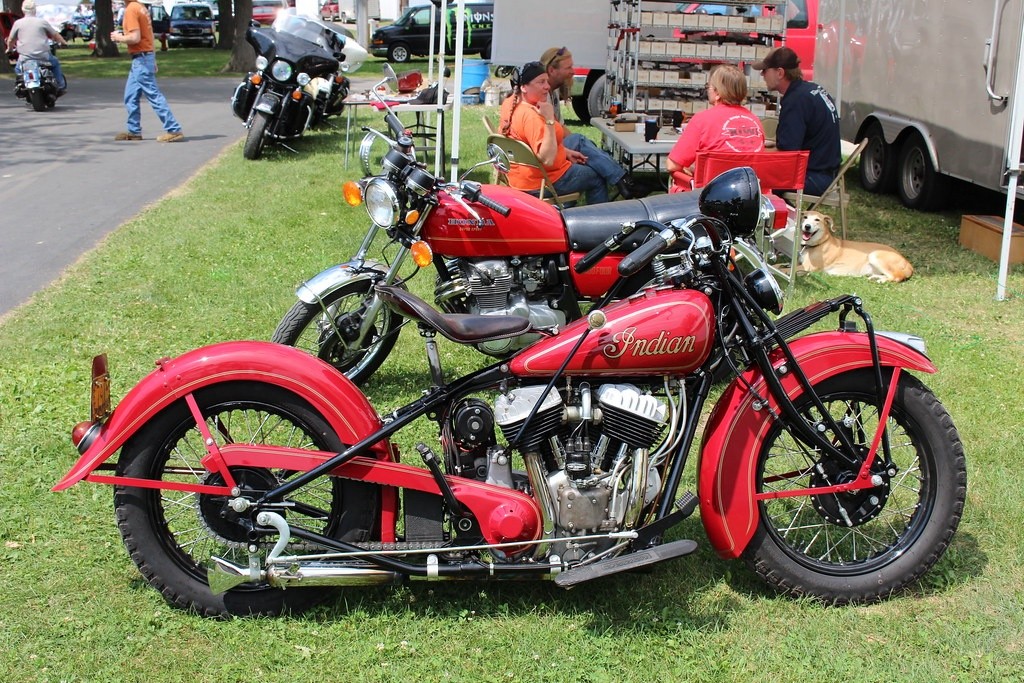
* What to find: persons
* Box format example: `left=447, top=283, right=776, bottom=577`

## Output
left=753, top=46, right=843, bottom=198
left=112, top=2, right=125, bottom=28
left=540, top=46, right=574, bottom=126
left=111, top=0, right=184, bottom=142
left=74, top=5, right=96, bottom=32
left=5, top=0, right=69, bottom=94
left=666, top=66, right=765, bottom=174
left=497, top=64, right=658, bottom=207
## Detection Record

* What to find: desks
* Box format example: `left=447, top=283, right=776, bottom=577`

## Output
left=341, top=93, right=454, bottom=178
left=590, top=116, right=777, bottom=199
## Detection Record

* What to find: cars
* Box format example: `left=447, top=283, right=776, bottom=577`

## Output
left=252, top=0, right=295, bottom=26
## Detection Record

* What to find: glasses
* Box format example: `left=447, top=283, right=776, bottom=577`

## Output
left=520, top=61, right=544, bottom=75
left=704, top=81, right=716, bottom=91
left=547, top=47, right=567, bottom=65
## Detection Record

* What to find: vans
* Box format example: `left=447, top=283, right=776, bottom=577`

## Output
left=320, top=0, right=380, bottom=24
left=151, top=2, right=219, bottom=49
left=371, top=2, right=494, bottom=61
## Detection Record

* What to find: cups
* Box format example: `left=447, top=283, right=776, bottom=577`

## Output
left=673, top=110, right=683, bottom=128
left=645, top=121, right=658, bottom=142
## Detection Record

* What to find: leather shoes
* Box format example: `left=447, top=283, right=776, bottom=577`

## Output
left=615, top=173, right=651, bottom=199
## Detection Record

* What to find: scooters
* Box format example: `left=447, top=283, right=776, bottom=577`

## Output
left=64, top=7, right=123, bottom=43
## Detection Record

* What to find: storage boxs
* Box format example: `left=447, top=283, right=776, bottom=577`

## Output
left=606, top=10, right=783, bottom=115
left=959, top=214, right=1024, bottom=267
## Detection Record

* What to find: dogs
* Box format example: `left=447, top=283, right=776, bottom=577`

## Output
left=771, top=211, right=914, bottom=284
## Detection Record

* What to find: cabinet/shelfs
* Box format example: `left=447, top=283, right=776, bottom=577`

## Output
left=601, top=1, right=791, bottom=177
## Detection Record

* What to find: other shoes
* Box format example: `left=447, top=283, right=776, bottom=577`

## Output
left=115, top=131, right=143, bottom=140
left=14, top=77, right=24, bottom=89
left=58, top=86, right=67, bottom=92
left=158, top=132, right=184, bottom=141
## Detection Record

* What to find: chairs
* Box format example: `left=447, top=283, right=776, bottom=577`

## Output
left=756, top=115, right=782, bottom=142
left=198, top=12, right=207, bottom=19
left=486, top=135, right=578, bottom=209
left=669, top=149, right=810, bottom=301
left=179, top=12, right=188, bottom=18
left=778, top=138, right=872, bottom=240
left=482, top=110, right=501, bottom=185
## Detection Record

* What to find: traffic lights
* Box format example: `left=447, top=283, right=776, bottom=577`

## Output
left=157, top=32, right=169, bottom=51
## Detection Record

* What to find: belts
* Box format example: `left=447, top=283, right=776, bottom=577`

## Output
left=132, top=51, right=153, bottom=59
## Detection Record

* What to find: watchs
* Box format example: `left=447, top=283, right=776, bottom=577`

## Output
left=545, top=120, right=554, bottom=124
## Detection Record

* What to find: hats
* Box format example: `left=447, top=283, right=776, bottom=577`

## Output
left=21, top=0, right=35, bottom=11
left=751, top=46, right=800, bottom=70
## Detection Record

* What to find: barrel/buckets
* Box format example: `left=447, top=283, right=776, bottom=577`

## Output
left=484, top=86, right=500, bottom=106
left=461, top=58, right=492, bottom=102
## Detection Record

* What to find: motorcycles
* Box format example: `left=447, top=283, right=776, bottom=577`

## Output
left=231, top=8, right=372, bottom=159
left=270, top=63, right=790, bottom=384
left=3, top=35, right=69, bottom=112
left=49, top=164, right=971, bottom=627
